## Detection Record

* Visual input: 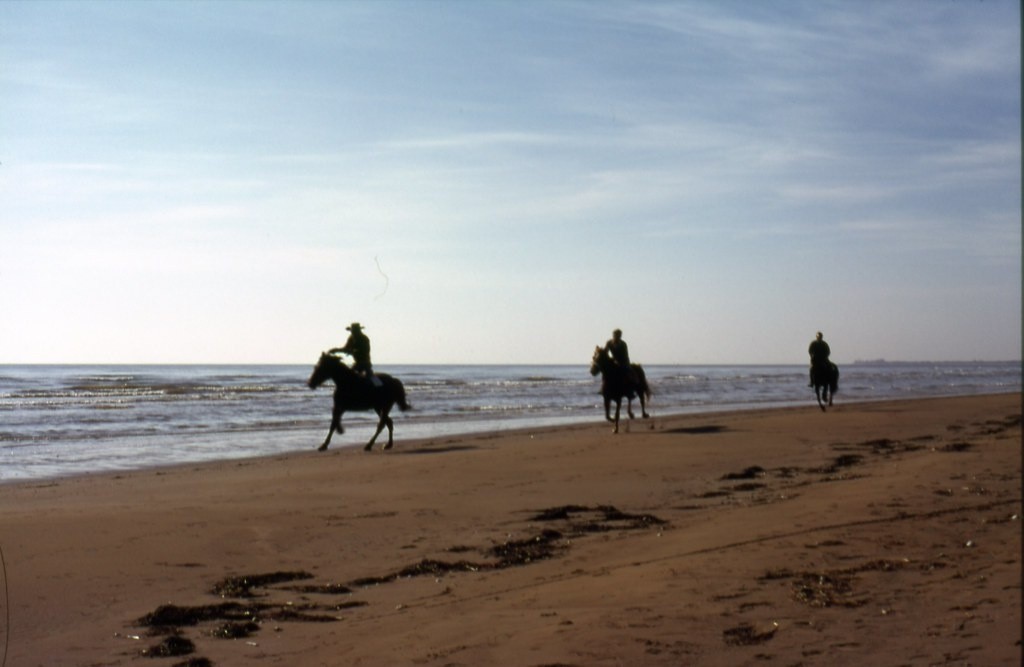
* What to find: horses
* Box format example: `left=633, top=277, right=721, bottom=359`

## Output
left=807, top=345, right=840, bottom=412
left=306, top=352, right=412, bottom=453
left=589, top=345, right=652, bottom=433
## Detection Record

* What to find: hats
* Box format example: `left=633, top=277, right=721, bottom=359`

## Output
left=346, top=322, right=365, bottom=330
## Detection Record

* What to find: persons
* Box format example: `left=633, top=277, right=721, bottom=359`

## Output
left=597, top=329, right=637, bottom=401
left=807, top=332, right=830, bottom=389
left=327, top=323, right=374, bottom=412
